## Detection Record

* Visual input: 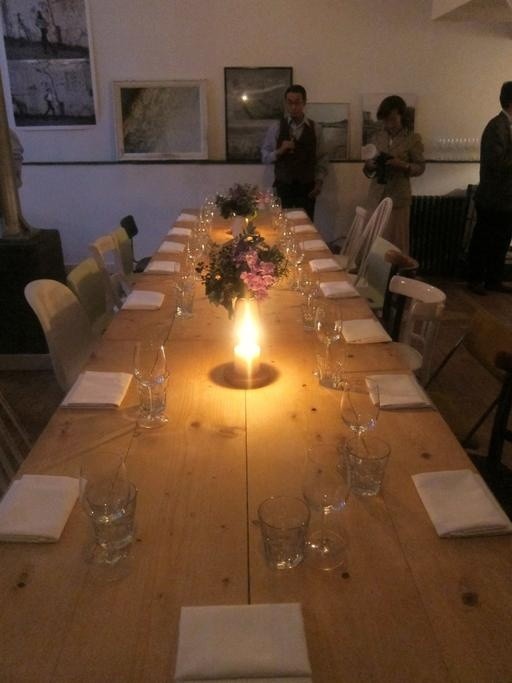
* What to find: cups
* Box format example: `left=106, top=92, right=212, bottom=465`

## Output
left=87, top=478, right=138, bottom=552
left=347, top=434, right=392, bottom=498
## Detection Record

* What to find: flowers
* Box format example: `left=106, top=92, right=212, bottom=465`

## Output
left=215, top=184, right=273, bottom=219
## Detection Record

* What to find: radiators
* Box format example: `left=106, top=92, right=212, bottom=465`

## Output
left=411, top=196, right=469, bottom=269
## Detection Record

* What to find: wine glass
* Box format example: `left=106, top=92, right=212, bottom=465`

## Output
left=128, top=186, right=383, bottom=573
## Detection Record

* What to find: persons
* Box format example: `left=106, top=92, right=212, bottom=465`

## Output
left=466, top=82, right=511, bottom=295
left=43, top=89, right=58, bottom=120
left=261, top=84, right=329, bottom=221
left=36, top=11, right=59, bottom=54
left=363, top=95, right=425, bottom=256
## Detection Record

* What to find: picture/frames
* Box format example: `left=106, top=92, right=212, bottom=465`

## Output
left=113, top=80, right=207, bottom=159
left=303, top=100, right=350, bottom=163
left=1, top=0, right=99, bottom=130
left=225, top=66, right=294, bottom=164
